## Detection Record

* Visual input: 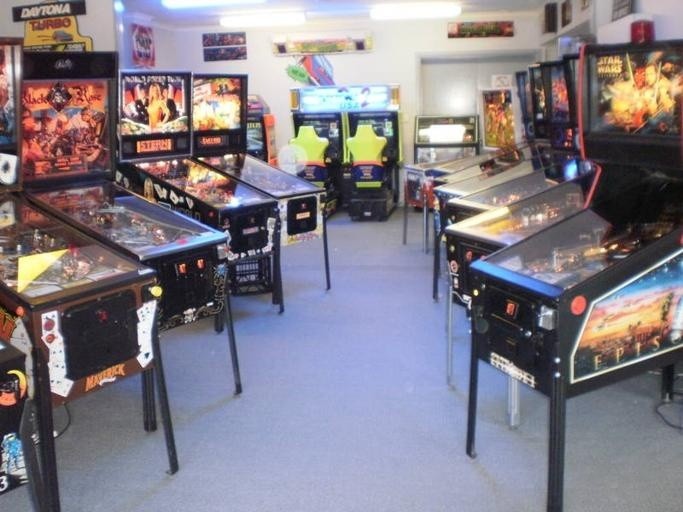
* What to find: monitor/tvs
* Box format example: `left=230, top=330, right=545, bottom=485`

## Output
left=539, top=59, right=573, bottom=150
left=562, top=53, right=579, bottom=150
left=117, top=69, right=192, bottom=162
left=479, top=88, right=519, bottom=152
left=516, top=70, right=536, bottom=139
left=20, top=51, right=117, bottom=190
left=527, top=64, right=549, bottom=139
left=192, top=72, right=248, bottom=156
left=0, top=35, right=25, bottom=195
left=290, top=111, right=346, bottom=164
left=578, top=38, right=683, bottom=168
left=346, top=111, right=404, bottom=166
left=247, top=114, right=278, bottom=164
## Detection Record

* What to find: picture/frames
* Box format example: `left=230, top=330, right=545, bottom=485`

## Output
left=610, top=0, right=632, bottom=21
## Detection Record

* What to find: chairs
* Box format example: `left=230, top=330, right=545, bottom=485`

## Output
left=345, top=123, right=387, bottom=189
left=287, top=125, right=330, bottom=188
